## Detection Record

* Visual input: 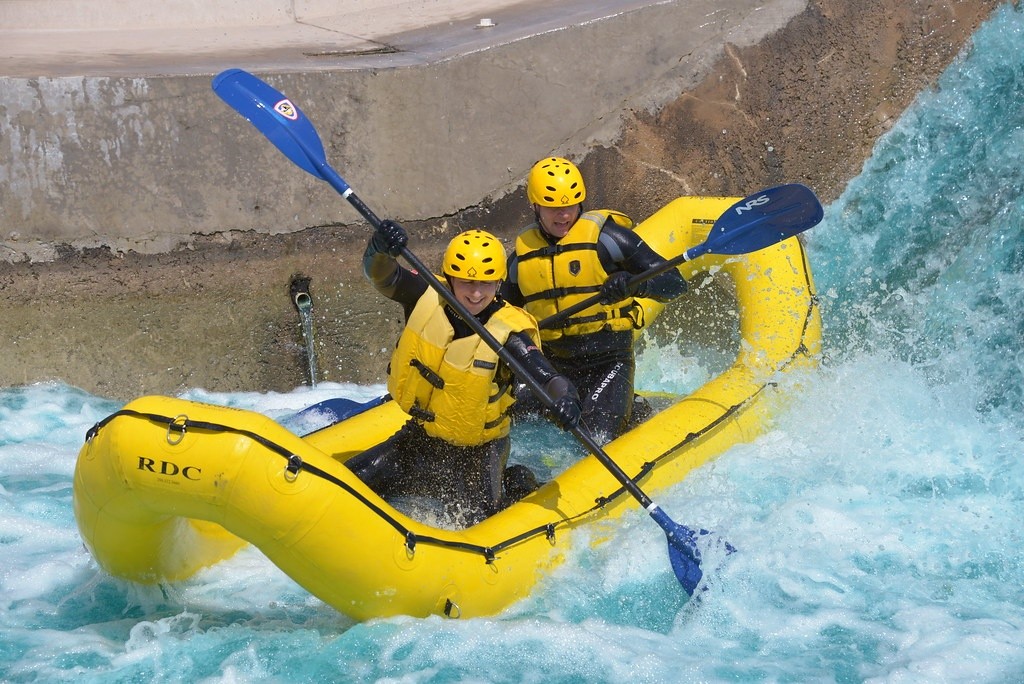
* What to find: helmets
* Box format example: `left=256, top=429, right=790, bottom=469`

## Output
left=526, top=157, right=587, bottom=207
left=443, top=228, right=507, bottom=282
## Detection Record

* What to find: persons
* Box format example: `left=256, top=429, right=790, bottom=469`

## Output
left=496, top=156, right=689, bottom=445
left=341, top=219, right=582, bottom=532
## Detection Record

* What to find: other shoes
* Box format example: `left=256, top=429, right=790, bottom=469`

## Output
left=504, top=465, right=539, bottom=491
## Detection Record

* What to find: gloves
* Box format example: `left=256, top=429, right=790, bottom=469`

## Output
left=551, top=396, right=581, bottom=432
left=600, top=271, right=641, bottom=306
left=372, top=220, right=408, bottom=259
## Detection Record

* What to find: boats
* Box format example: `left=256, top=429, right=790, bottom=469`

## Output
left=68, top=190, right=825, bottom=624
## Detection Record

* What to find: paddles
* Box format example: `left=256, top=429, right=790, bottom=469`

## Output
left=212, top=67, right=736, bottom=596
left=296, top=184, right=824, bottom=421
